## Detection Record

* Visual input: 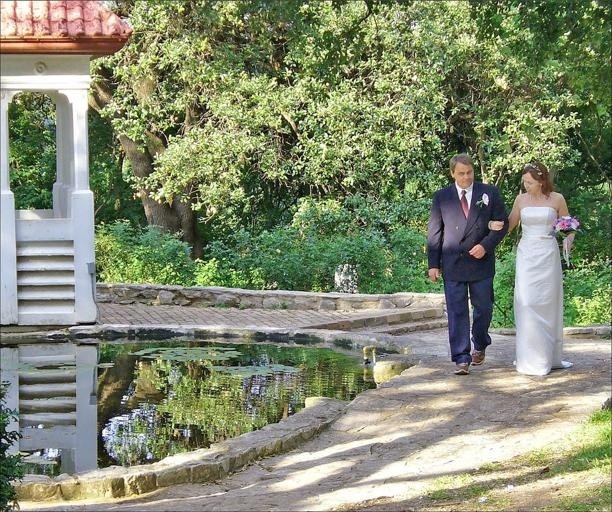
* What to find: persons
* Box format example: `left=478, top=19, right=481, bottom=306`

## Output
left=486, top=161, right=575, bottom=376
left=424, top=153, right=510, bottom=375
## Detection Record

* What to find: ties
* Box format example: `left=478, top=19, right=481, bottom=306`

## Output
left=460, top=190, right=468, bottom=219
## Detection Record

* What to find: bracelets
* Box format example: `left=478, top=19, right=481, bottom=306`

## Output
left=487, top=220, right=493, bottom=230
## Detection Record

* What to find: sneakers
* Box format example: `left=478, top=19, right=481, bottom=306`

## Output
left=454, top=362, right=470, bottom=374
left=471, top=350, right=485, bottom=366
